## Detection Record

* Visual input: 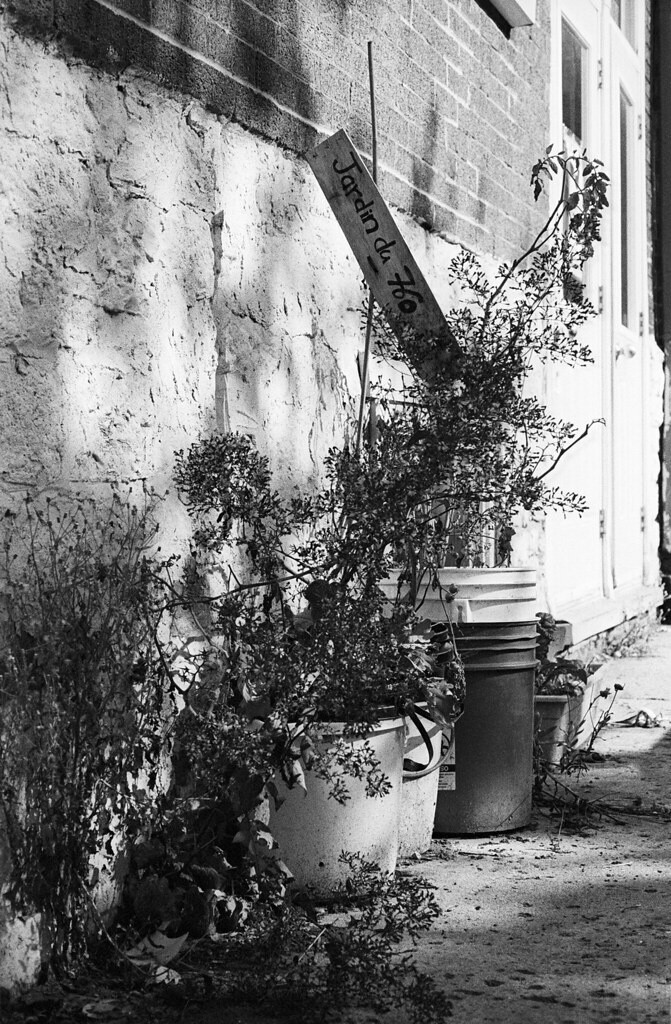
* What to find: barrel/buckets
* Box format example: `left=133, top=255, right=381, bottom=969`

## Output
left=370, top=559, right=541, bottom=839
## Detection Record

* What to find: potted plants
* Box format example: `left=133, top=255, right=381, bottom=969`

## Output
left=169, top=141, right=610, bottom=911
